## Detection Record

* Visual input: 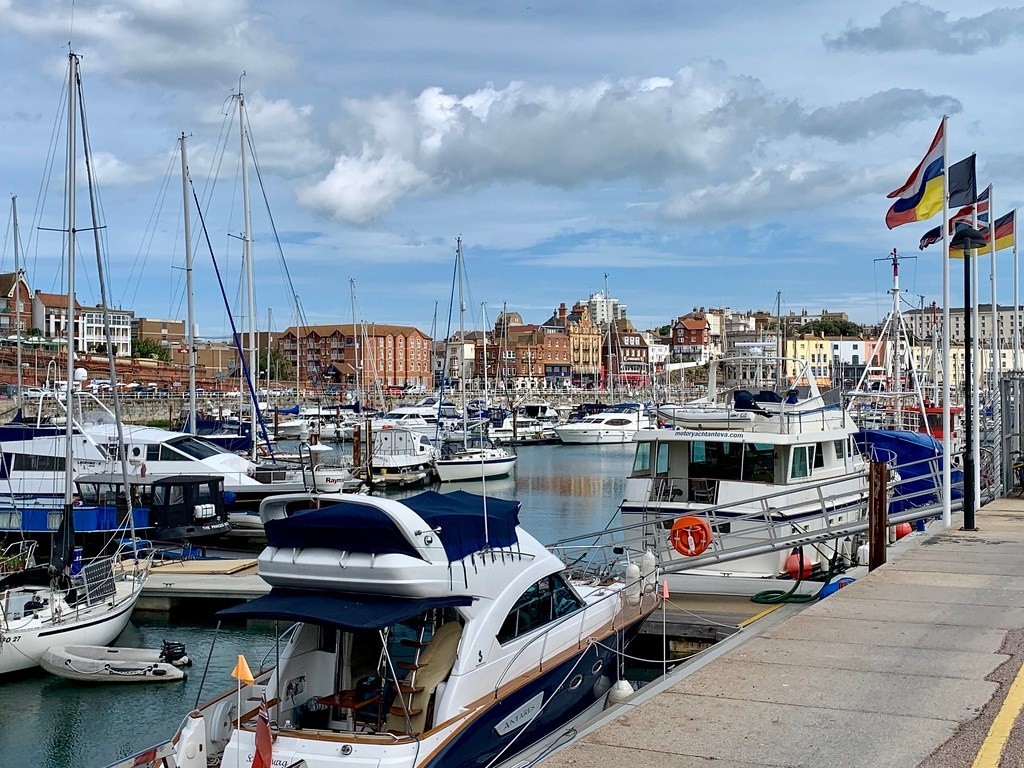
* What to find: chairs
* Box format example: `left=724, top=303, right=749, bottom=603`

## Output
left=355, top=678, right=396, bottom=733
left=655, top=478, right=683, bottom=501
left=691, top=479, right=714, bottom=504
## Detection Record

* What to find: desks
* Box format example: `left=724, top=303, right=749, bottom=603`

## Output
left=316, top=690, right=384, bottom=734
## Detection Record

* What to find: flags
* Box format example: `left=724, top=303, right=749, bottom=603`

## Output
left=949, top=154, right=976, bottom=209
left=251, top=693, right=272, bottom=768
left=949, top=210, right=1014, bottom=259
left=885, top=119, right=943, bottom=230
left=918, top=186, right=988, bottom=250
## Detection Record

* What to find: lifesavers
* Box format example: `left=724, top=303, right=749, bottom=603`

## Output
left=674, top=526, right=706, bottom=553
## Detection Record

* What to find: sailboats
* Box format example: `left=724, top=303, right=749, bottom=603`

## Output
left=0, top=35, right=149, bottom=676
left=0, top=71, right=985, bottom=540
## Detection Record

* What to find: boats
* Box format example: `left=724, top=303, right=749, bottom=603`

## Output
left=40, top=639, right=194, bottom=682
left=100, top=440, right=667, bottom=765
left=542, top=291, right=902, bottom=641
left=844, top=247, right=1003, bottom=529
